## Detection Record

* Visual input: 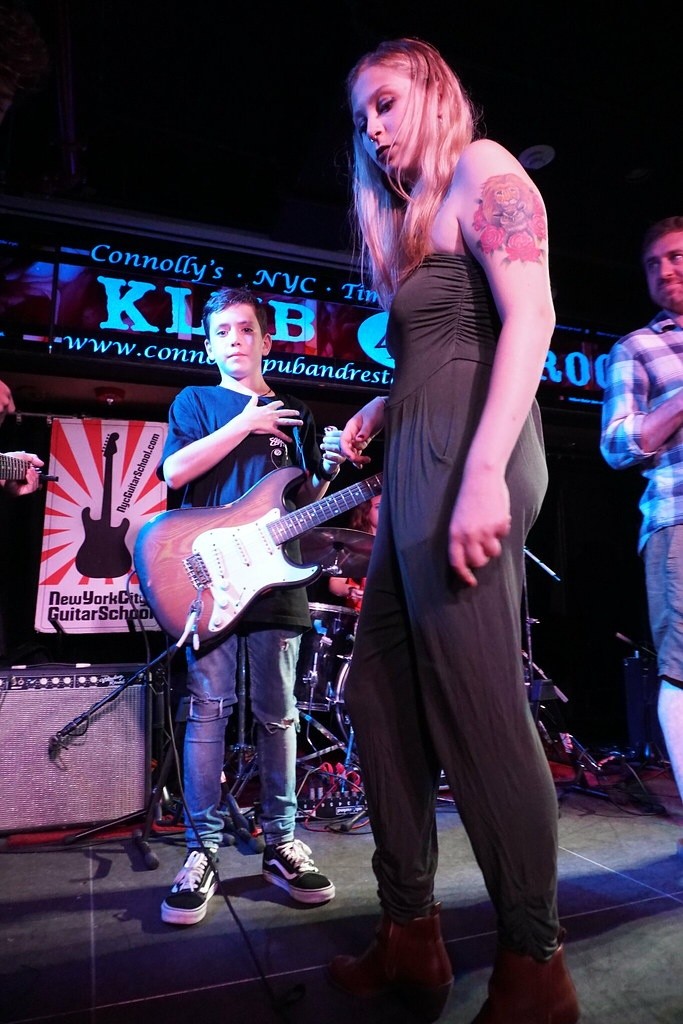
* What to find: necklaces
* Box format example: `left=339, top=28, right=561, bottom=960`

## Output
left=258, top=389, right=271, bottom=397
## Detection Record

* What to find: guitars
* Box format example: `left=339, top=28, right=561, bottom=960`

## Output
left=0, top=453, right=60, bottom=483
left=133, top=464, right=385, bottom=646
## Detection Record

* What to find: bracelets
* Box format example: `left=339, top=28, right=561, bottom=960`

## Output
left=319, top=457, right=340, bottom=481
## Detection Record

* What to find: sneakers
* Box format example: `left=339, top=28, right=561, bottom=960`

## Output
left=160, top=847, right=220, bottom=923
left=261, top=839, right=335, bottom=903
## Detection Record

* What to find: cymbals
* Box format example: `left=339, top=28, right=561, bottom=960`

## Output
left=299, top=525, right=376, bottom=579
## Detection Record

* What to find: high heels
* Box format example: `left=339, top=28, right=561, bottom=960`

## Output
left=470, top=925, right=581, bottom=1024
left=325, top=903, right=455, bottom=1022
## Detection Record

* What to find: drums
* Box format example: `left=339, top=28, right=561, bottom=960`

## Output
left=293, top=601, right=360, bottom=712
left=335, top=652, right=450, bottom=792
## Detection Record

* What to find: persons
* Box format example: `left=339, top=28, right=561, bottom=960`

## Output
left=156, top=286, right=347, bottom=924
left=328, top=40, right=581, bottom=1024
left=329, top=494, right=389, bottom=612
left=601, top=216, right=683, bottom=855
left=0, top=380, right=44, bottom=496
